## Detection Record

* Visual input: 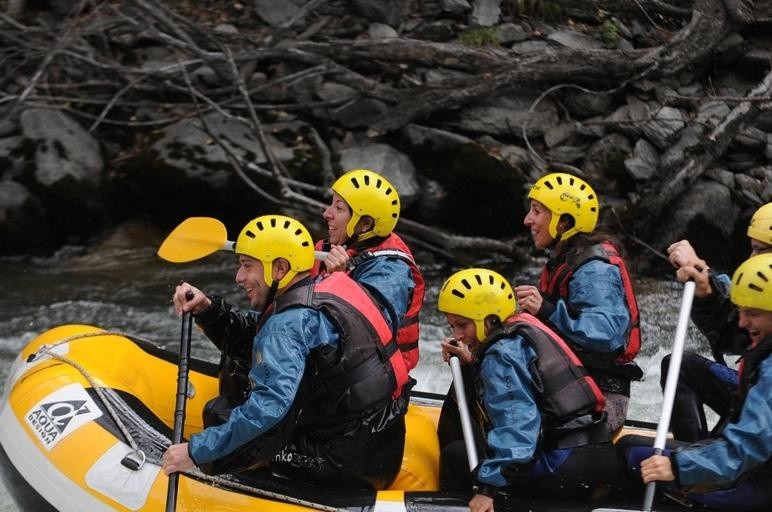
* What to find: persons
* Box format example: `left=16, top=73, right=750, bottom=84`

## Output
left=310, top=170, right=424, bottom=489
left=614, top=201, right=771, bottom=512
left=161, top=215, right=407, bottom=511
left=437, top=269, right=618, bottom=511
left=513, top=173, right=640, bottom=433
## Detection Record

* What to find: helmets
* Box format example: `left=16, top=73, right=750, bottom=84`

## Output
left=526, top=173, right=600, bottom=241
left=332, top=169, right=401, bottom=242
left=731, top=254, right=771, bottom=313
left=236, top=214, right=316, bottom=290
left=747, top=201, right=771, bottom=245
left=438, top=267, right=517, bottom=345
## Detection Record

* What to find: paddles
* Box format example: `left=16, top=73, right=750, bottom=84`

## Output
left=157, top=217, right=347, bottom=264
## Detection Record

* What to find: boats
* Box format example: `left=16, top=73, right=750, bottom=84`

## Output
left=0, top=324, right=713, bottom=512
